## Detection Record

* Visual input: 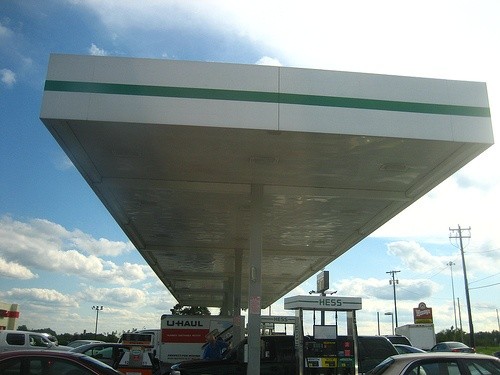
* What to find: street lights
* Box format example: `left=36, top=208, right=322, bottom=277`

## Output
left=92, top=305, right=104, bottom=340
left=385, top=312, right=394, bottom=335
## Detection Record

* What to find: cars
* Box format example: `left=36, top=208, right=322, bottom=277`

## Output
left=382, top=335, right=439, bottom=375
left=363, top=352, right=500, bottom=375
left=0, top=328, right=162, bottom=375
left=430, top=340, right=476, bottom=366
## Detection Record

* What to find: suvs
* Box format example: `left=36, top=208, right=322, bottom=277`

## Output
left=170, top=334, right=416, bottom=375
